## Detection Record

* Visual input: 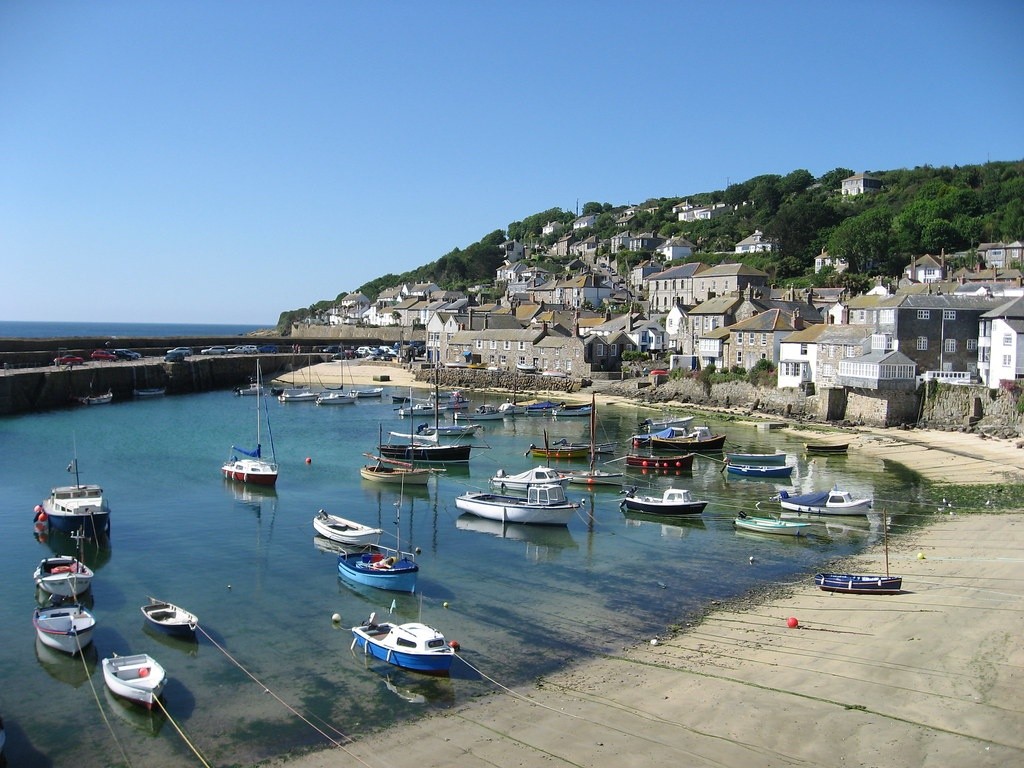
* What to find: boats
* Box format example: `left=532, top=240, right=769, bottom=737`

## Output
left=32, top=526, right=94, bottom=598
left=140, top=595, right=198, bottom=639
left=349, top=363, right=592, bottom=437
left=32, top=593, right=96, bottom=656
left=78, top=388, right=167, bottom=406
left=352, top=611, right=455, bottom=675
left=314, top=471, right=418, bottom=594
left=43, top=430, right=111, bottom=542
left=530, top=415, right=873, bottom=516
left=735, top=511, right=812, bottom=537
left=143, top=617, right=199, bottom=658
left=625, top=510, right=708, bottom=542
left=34, top=632, right=99, bottom=691
left=454, top=481, right=580, bottom=526
left=457, top=513, right=579, bottom=563
left=101, top=670, right=166, bottom=739
left=102, top=652, right=168, bottom=713
left=814, top=508, right=903, bottom=594
left=314, top=534, right=419, bottom=623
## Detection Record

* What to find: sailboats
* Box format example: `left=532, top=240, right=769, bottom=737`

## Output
left=225, top=479, right=279, bottom=593
left=221, top=358, right=279, bottom=486
left=234, top=341, right=359, bottom=405
left=361, top=334, right=493, bottom=486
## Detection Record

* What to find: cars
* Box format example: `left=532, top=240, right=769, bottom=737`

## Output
left=53, top=342, right=425, bottom=366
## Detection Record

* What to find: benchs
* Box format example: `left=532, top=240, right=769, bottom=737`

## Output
left=147, top=608, right=176, bottom=616
left=118, top=662, right=150, bottom=671
left=365, top=628, right=390, bottom=635
